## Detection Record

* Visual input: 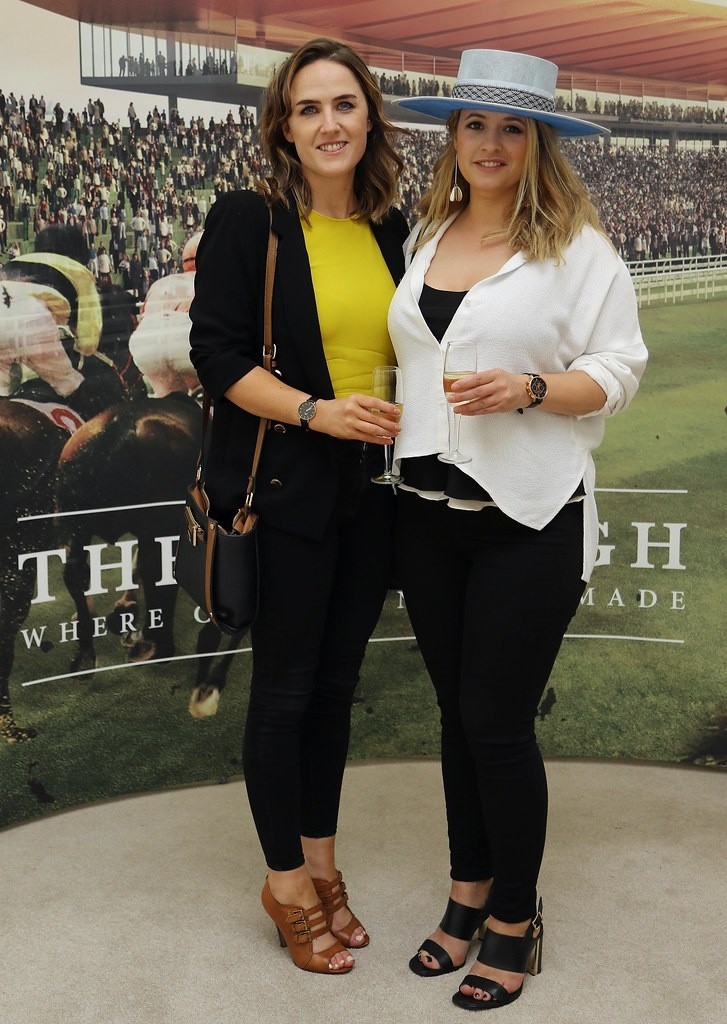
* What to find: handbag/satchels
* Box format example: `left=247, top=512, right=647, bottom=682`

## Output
left=173, top=483, right=261, bottom=634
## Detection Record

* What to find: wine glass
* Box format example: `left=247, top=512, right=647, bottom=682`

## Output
left=370, top=365, right=405, bottom=485
left=437, top=339, right=478, bottom=465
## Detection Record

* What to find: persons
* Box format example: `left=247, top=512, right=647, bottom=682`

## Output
left=1, top=52, right=727, bottom=526
left=385, top=50, right=651, bottom=1009
left=195, top=37, right=414, bottom=976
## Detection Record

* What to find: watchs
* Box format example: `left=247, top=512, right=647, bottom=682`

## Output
left=515, top=372, right=547, bottom=415
left=298, top=394, right=320, bottom=429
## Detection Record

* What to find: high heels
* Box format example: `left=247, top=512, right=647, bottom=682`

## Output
left=260, top=874, right=352, bottom=973
left=452, top=896, right=544, bottom=1010
left=409, top=896, right=490, bottom=977
left=312, top=868, right=369, bottom=948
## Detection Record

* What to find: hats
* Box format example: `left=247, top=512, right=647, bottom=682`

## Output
left=391, top=49, right=611, bottom=139
